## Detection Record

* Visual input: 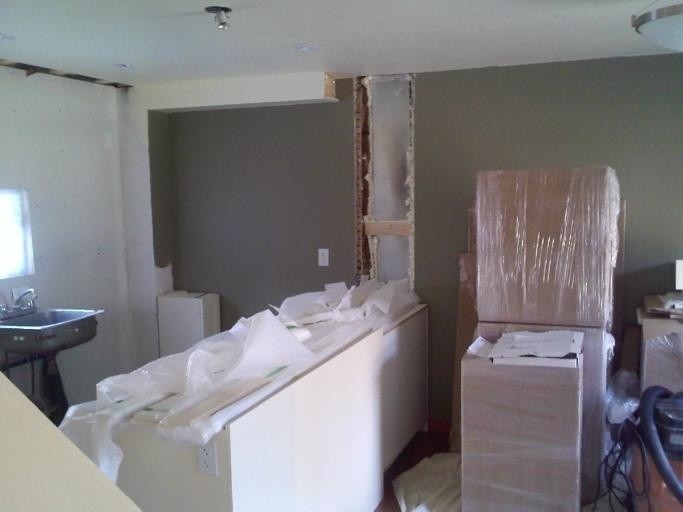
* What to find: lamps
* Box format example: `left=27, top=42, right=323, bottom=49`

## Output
left=629, top=3, right=683, bottom=58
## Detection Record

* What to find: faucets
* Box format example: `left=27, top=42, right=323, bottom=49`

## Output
left=13, top=288, right=38, bottom=311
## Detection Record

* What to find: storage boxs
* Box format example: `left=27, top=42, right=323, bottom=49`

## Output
left=472, top=323, right=613, bottom=507
left=463, top=337, right=589, bottom=511
left=472, top=166, right=618, bottom=330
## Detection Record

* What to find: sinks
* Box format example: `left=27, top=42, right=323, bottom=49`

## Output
left=1, top=306, right=107, bottom=355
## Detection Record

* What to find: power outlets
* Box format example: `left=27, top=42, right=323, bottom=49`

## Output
left=195, top=441, right=218, bottom=479
